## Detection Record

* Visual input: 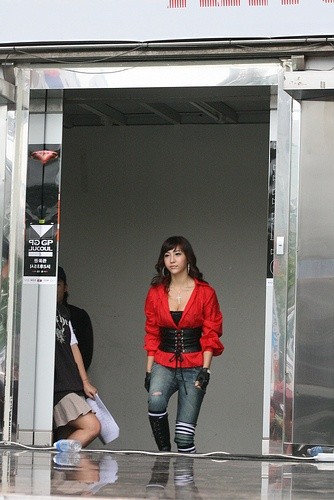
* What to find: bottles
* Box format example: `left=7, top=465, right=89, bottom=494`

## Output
left=306, top=446, right=334, bottom=456
left=53, top=439, right=82, bottom=453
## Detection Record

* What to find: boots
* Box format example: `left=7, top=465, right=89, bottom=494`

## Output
left=146, top=455, right=170, bottom=489
left=148, top=409, right=171, bottom=452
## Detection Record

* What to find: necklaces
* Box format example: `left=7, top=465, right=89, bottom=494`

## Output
left=176, top=293, right=183, bottom=305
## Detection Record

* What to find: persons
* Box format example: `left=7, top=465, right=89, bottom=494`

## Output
left=51, top=452, right=100, bottom=496
left=145, top=458, right=202, bottom=499
left=53, top=265, right=101, bottom=449
left=143, top=235, right=224, bottom=453
